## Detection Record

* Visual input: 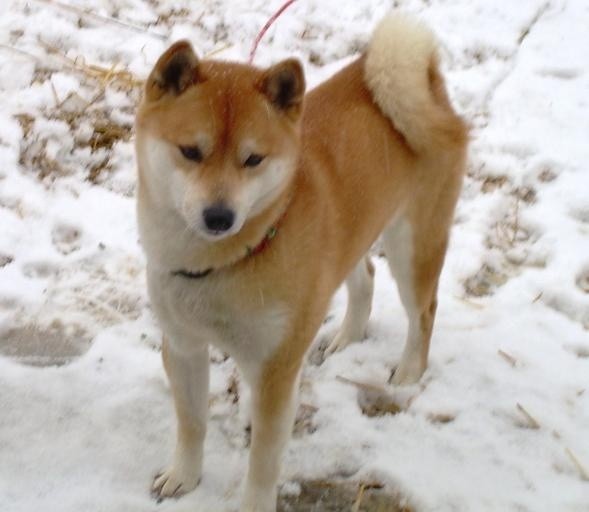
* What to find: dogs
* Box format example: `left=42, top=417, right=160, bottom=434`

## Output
left=133, top=8, right=469, bottom=512
